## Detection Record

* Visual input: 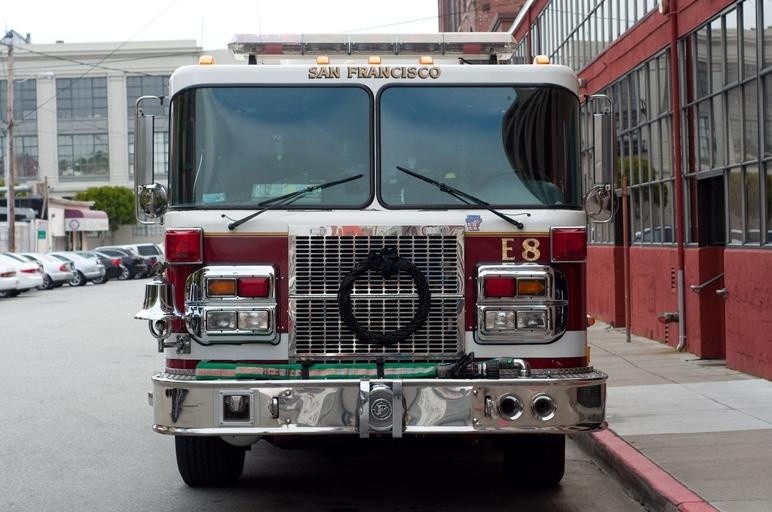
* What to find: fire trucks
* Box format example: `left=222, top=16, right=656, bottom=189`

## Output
left=134, top=29, right=617, bottom=488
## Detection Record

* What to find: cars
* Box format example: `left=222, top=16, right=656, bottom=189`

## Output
left=0, top=243, right=166, bottom=297
